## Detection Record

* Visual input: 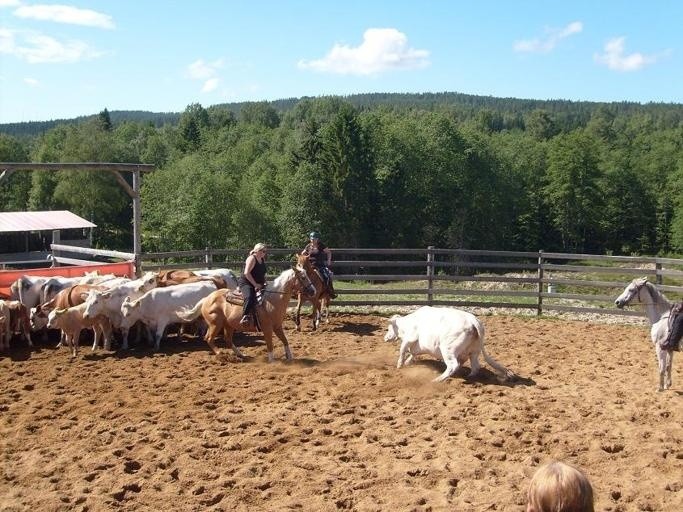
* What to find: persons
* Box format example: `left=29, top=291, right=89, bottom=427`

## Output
left=238, top=242, right=267, bottom=325
left=301, top=232, right=337, bottom=299
left=527, top=460, right=594, bottom=512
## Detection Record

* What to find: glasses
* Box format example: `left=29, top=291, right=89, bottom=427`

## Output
left=260, top=250, right=266, bottom=253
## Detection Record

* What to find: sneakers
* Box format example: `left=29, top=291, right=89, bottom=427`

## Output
left=239, top=316, right=250, bottom=327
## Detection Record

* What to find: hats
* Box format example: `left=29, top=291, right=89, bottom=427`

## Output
left=310, top=232, right=318, bottom=240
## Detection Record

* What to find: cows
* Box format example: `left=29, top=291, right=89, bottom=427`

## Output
left=138, top=267, right=239, bottom=293
left=0, top=300, right=11, bottom=349
left=0, top=290, right=11, bottom=301
left=46, top=299, right=110, bottom=359
left=117, top=280, right=217, bottom=352
left=83, top=278, right=156, bottom=351
left=5, top=300, right=33, bottom=349
left=381, top=305, right=516, bottom=385
left=156, top=269, right=227, bottom=331
left=39, top=270, right=108, bottom=302
left=29, top=284, right=109, bottom=349
left=10, top=270, right=99, bottom=326
left=159, top=270, right=196, bottom=279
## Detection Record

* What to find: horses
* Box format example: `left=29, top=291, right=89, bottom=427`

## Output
left=173, top=262, right=317, bottom=362
left=293, top=252, right=339, bottom=332
left=613, top=276, right=683, bottom=394
